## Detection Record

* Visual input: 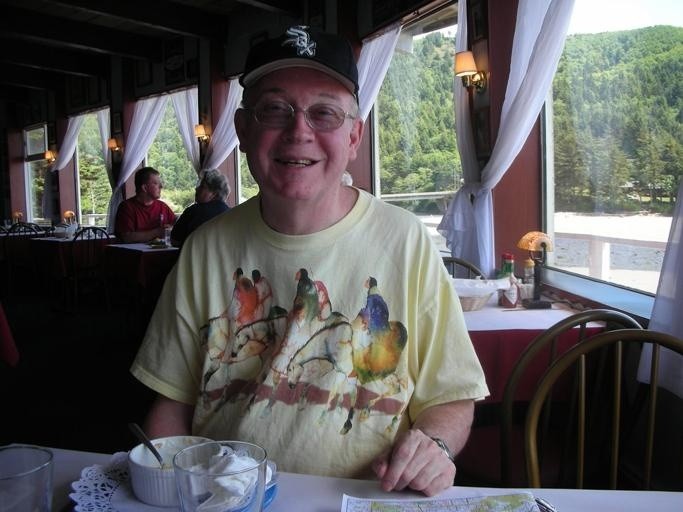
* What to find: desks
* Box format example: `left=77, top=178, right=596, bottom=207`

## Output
left=456, top=308, right=612, bottom=410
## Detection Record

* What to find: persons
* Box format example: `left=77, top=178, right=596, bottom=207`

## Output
left=114, top=167, right=176, bottom=243
left=130, top=24, right=493, bottom=497
left=169, top=167, right=232, bottom=248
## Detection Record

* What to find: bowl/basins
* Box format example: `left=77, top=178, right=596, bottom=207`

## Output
left=127, top=436, right=223, bottom=508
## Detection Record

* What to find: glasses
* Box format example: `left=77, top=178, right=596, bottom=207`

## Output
left=239, top=97, right=358, bottom=133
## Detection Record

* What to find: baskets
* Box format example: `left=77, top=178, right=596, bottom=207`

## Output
left=458, top=292, right=495, bottom=312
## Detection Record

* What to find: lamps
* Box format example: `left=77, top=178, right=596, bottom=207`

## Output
left=454, top=50, right=488, bottom=94
left=107, top=136, right=124, bottom=155
left=517, top=231, right=551, bottom=309
left=44, top=150, right=58, bottom=167
left=194, top=123, right=211, bottom=146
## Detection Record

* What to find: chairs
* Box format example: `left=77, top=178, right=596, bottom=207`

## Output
left=527, top=328, right=683, bottom=491
left=497, top=310, right=650, bottom=484
left=443, top=256, right=486, bottom=279
left=6, top=221, right=111, bottom=323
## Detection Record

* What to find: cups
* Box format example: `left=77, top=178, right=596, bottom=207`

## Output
left=1, top=443, right=53, bottom=512
left=173, top=440, right=268, bottom=512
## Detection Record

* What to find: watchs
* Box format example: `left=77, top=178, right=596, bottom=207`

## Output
left=432, top=437, right=452, bottom=459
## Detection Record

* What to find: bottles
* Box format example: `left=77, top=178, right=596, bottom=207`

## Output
left=498, top=252, right=537, bottom=308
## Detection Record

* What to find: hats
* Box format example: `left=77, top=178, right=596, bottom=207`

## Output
left=238, top=24, right=360, bottom=101
